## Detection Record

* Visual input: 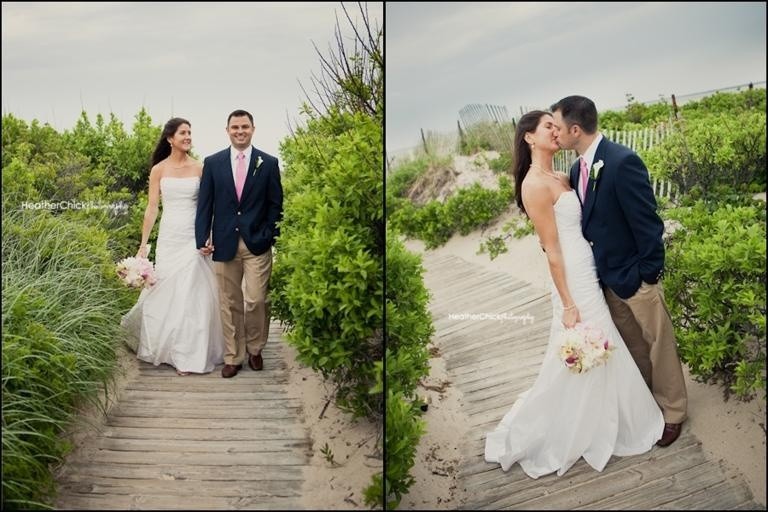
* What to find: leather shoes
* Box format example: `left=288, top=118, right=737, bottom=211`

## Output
left=248, top=354, right=262, bottom=371
left=221, top=363, right=242, bottom=378
left=655, top=423, right=680, bottom=447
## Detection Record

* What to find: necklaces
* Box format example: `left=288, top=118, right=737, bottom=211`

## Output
left=530, top=160, right=572, bottom=190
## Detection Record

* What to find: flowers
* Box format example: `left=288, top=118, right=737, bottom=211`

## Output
left=559, top=323, right=618, bottom=376
left=114, top=256, right=159, bottom=292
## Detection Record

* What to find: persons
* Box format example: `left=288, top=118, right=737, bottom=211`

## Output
left=195, top=108, right=285, bottom=377
left=551, top=96, right=688, bottom=448
left=115, top=118, right=226, bottom=376
left=483, top=110, right=667, bottom=480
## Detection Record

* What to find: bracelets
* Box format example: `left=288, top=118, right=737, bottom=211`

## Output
left=139, top=245, right=147, bottom=248
left=562, top=304, right=576, bottom=311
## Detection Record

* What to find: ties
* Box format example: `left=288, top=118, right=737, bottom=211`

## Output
left=579, top=159, right=588, bottom=198
left=236, top=152, right=245, bottom=202
